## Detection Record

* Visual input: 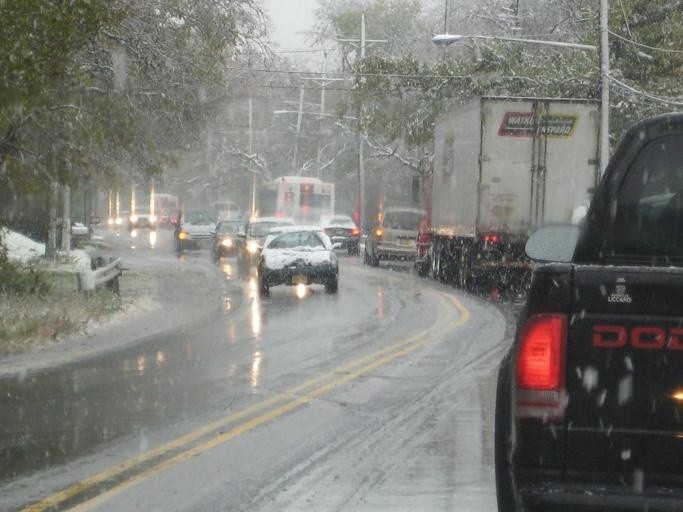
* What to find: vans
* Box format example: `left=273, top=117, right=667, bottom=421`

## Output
left=365, top=208, right=426, bottom=267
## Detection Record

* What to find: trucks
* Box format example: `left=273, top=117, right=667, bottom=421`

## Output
left=412, top=94, right=602, bottom=291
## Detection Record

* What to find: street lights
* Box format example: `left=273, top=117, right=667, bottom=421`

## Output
left=274, top=109, right=366, bottom=235
left=434, top=33, right=611, bottom=184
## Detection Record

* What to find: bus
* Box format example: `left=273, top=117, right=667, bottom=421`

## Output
left=258, top=176, right=335, bottom=225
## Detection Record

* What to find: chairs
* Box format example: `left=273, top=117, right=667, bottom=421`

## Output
left=221, top=225, right=232, bottom=232
left=656, top=191, right=683, bottom=251
left=269, top=240, right=286, bottom=246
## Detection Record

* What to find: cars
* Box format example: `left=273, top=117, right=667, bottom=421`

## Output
left=209, top=220, right=248, bottom=265
left=174, top=224, right=216, bottom=250
left=316, top=215, right=360, bottom=255
left=257, top=225, right=342, bottom=296
left=128, top=217, right=155, bottom=232
left=208, top=201, right=242, bottom=222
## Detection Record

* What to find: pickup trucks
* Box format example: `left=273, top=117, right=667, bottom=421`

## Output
left=494, top=108, right=683, bottom=512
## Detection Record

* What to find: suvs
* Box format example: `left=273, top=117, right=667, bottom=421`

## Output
left=235, top=217, right=297, bottom=278
